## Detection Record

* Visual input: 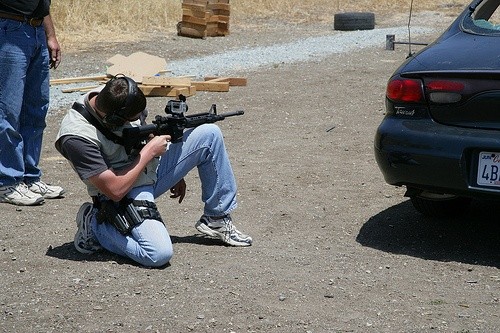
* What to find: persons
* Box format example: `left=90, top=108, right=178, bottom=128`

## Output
left=55, top=77, right=253, bottom=267
left=0, top=0, right=65, bottom=206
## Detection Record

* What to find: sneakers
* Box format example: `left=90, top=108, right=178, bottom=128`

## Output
left=0, top=183, right=44, bottom=205
left=25, top=180, right=64, bottom=198
left=195, top=214, right=253, bottom=246
left=74, top=202, right=103, bottom=254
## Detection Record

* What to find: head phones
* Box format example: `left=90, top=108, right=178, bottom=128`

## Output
left=106, top=77, right=137, bottom=127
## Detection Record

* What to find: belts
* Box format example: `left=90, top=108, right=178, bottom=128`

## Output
left=0, top=13, right=44, bottom=27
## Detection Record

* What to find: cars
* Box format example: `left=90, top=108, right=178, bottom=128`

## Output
left=371, top=0, right=500, bottom=219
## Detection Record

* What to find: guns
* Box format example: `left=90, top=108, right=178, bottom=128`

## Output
left=123, top=94, right=244, bottom=157
